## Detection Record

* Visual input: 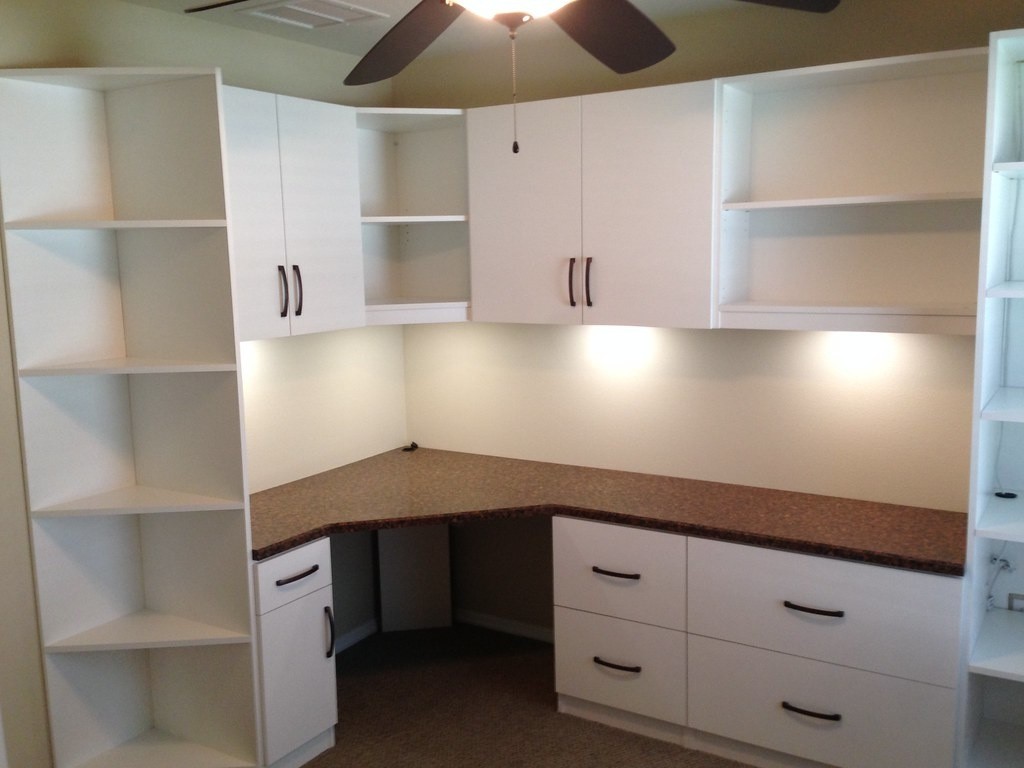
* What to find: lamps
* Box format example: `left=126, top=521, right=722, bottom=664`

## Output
left=445, top=0, right=577, bottom=153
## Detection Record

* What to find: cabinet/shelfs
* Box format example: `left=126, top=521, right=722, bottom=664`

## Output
left=0, top=29, right=1024, bottom=768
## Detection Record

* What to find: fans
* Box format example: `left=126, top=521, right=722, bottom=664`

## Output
left=186, top=0, right=842, bottom=87
